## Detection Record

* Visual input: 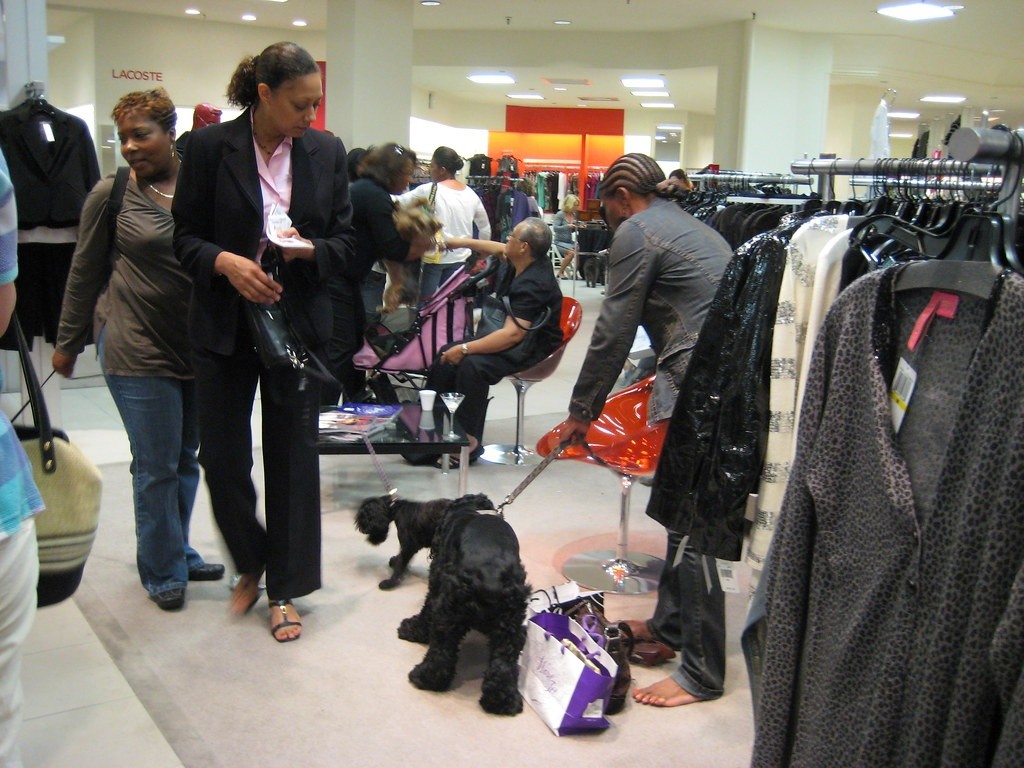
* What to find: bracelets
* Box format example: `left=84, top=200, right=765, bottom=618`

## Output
left=441, top=240, right=453, bottom=252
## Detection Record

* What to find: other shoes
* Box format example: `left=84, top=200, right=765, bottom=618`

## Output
left=556, top=274, right=568, bottom=280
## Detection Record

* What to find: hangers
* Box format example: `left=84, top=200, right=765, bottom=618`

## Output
left=523, top=164, right=604, bottom=176
left=698, top=122, right=1024, bottom=303
left=466, top=173, right=527, bottom=190
left=24, top=82, right=55, bottom=119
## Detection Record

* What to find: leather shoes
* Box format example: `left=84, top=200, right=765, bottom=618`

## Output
left=155, top=587, right=183, bottom=608
left=189, top=564, right=224, bottom=581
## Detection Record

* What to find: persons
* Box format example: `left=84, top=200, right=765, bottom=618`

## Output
left=670, top=169, right=693, bottom=187
left=319, top=141, right=445, bottom=405
left=52, top=88, right=224, bottom=610
left=0, top=152, right=49, bottom=768
left=515, top=177, right=541, bottom=218
left=559, top=153, right=733, bottom=706
left=553, top=194, right=586, bottom=280
left=425, top=217, right=563, bottom=470
left=170, top=41, right=357, bottom=644
left=390, top=145, right=491, bottom=308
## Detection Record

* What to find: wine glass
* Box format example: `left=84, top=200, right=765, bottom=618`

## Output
left=441, top=392, right=465, bottom=441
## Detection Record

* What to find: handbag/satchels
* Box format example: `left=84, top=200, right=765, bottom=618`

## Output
left=244, top=245, right=301, bottom=371
left=12, top=309, right=102, bottom=607
left=516, top=580, right=635, bottom=738
left=422, top=183, right=442, bottom=263
left=476, top=295, right=552, bottom=363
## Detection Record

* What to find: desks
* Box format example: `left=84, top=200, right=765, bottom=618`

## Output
left=317, top=400, right=472, bottom=499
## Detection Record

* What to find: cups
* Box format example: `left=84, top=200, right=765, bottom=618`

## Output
left=419, top=390, right=437, bottom=411
left=418, top=412, right=436, bottom=430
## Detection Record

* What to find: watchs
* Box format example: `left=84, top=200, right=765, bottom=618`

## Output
left=462, top=343, right=468, bottom=356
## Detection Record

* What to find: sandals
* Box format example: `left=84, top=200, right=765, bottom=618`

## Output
left=269, top=599, right=302, bottom=642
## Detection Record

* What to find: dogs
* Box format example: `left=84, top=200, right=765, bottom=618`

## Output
left=375, top=198, right=446, bottom=312
left=354, top=495, right=454, bottom=589
left=398, top=492, right=524, bottom=718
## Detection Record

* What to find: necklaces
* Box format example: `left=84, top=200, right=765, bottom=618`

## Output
left=253, top=129, right=273, bottom=155
left=143, top=177, right=174, bottom=199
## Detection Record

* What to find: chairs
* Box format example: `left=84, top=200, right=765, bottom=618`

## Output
left=533, top=371, right=675, bottom=596
left=474, top=295, right=584, bottom=465
left=547, top=226, right=579, bottom=279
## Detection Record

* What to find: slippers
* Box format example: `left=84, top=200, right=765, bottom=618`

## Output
left=433, top=445, right=485, bottom=469
